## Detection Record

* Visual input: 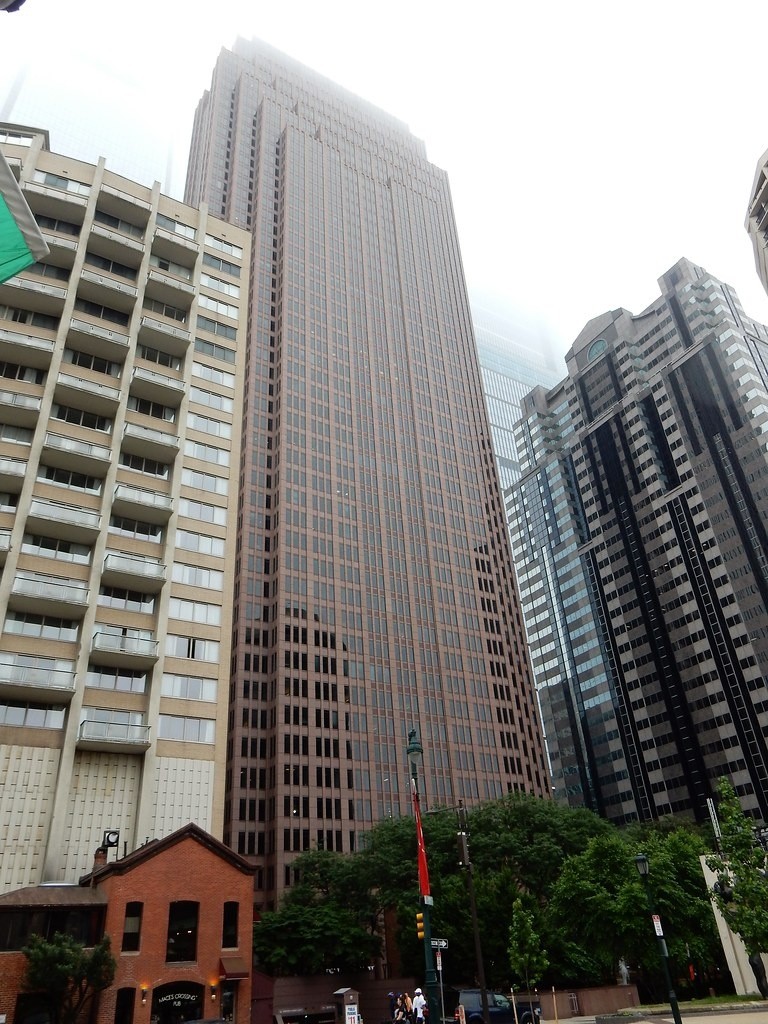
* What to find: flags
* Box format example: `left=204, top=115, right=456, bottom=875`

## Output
left=413, top=791, right=429, bottom=896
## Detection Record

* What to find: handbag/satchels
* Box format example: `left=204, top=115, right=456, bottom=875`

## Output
left=392, top=1016, right=407, bottom=1024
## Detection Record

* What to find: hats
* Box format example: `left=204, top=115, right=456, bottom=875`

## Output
left=414, top=988, right=422, bottom=993
left=387, top=992, right=394, bottom=996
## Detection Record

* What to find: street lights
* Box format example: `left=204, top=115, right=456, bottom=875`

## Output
left=406, top=727, right=443, bottom=1024
left=633, top=852, right=683, bottom=1024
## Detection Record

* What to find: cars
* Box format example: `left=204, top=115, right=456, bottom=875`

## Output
left=440, top=987, right=540, bottom=1024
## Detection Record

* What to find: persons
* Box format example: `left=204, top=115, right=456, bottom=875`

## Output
left=385, top=986, right=441, bottom=1024
left=396, top=998, right=406, bottom=1024
